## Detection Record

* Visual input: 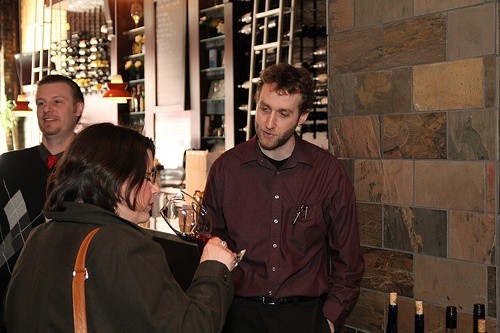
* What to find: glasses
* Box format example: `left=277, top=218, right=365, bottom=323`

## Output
left=145, top=170, right=157, bottom=184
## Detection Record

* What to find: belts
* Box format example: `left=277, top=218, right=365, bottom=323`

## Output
left=248, top=296, right=314, bottom=306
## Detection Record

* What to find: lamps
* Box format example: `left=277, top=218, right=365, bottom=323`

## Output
left=102, top=0, right=134, bottom=103
left=12, top=0, right=33, bottom=116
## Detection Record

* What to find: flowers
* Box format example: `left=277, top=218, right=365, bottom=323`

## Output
left=1, top=99, right=21, bottom=132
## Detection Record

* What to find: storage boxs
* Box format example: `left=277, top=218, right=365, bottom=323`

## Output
left=185, top=150, right=228, bottom=213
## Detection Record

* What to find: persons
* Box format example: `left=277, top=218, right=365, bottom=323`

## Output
left=195, top=63, right=366, bottom=333
left=0, top=75, right=85, bottom=309
left=0, top=122, right=247, bottom=333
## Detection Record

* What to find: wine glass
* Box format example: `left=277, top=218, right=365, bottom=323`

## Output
left=131, top=3, right=142, bottom=28
left=160, top=190, right=246, bottom=272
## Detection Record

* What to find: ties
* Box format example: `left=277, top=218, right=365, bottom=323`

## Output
left=47, top=156, right=56, bottom=168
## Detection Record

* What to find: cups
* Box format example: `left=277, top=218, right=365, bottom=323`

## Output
left=200, top=115, right=215, bottom=135
left=178, top=209, right=192, bottom=226
left=210, top=49, right=216, bottom=68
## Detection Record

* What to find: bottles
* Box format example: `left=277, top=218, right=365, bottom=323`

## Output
left=446, top=306, right=457, bottom=333
left=135, top=85, right=143, bottom=112
left=414, top=301, right=424, bottom=333
left=313, top=86, right=329, bottom=94
left=311, top=73, right=328, bottom=82
left=238, top=12, right=253, bottom=24
left=129, top=86, right=136, bottom=111
left=313, top=97, right=328, bottom=105
left=238, top=104, right=248, bottom=111
left=386, top=293, right=399, bottom=333
left=237, top=81, right=249, bottom=89
left=238, top=24, right=252, bottom=34
left=311, top=62, right=326, bottom=68
left=238, top=125, right=247, bottom=132
left=310, top=47, right=326, bottom=56
left=255, top=48, right=275, bottom=54
left=284, top=29, right=302, bottom=38
left=473, top=304, right=485, bottom=333
left=259, top=19, right=277, bottom=29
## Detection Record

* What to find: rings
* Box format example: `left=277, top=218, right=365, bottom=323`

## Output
left=222, top=240, right=228, bottom=248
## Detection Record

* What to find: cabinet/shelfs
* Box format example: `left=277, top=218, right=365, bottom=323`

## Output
left=105, top=0, right=144, bottom=137
left=138, top=217, right=210, bottom=258
left=188, top=0, right=328, bottom=217
